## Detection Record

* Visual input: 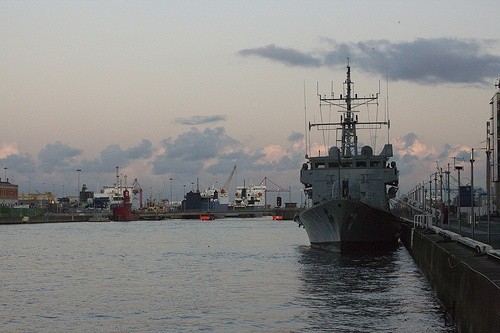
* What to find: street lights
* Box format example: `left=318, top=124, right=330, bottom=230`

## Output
left=407, top=138, right=492, bottom=241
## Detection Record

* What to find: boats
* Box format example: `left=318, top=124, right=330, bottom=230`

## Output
left=294, top=58, right=403, bottom=252
